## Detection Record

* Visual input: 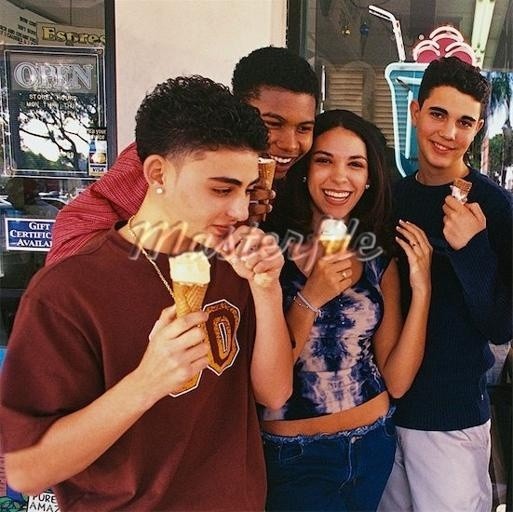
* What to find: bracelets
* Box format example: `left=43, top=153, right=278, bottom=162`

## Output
left=295, top=290, right=321, bottom=316
left=289, top=295, right=310, bottom=311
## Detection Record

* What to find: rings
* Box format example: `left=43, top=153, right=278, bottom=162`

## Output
left=341, top=270, right=347, bottom=280
left=410, top=241, right=419, bottom=249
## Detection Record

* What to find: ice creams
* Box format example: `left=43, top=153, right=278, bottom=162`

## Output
left=258, top=157, right=276, bottom=222
left=168, top=250, right=211, bottom=319
left=453, top=177, right=473, bottom=202
left=319, top=219, right=352, bottom=257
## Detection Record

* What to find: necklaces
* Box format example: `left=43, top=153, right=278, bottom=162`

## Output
left=127, top=214, right=197, bottom=302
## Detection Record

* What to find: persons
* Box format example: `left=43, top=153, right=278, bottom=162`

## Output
left=363, top=52, right=513, bottom=512
left=251, top=107, right=436, bottom=509
left=41, top=43, right=321, bottom=267
left=2, top=75, right=294, bottom=512
